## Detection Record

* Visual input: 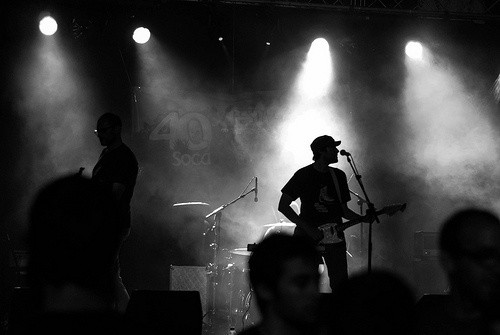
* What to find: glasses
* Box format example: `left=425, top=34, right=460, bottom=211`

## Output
left=92, top=125, right=115, bottom=135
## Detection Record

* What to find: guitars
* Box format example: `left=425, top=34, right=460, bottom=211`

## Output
left=294, top=201, right=409, bottom=246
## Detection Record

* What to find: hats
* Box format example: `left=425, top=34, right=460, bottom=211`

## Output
left=311, top=136, right=341, bottom=153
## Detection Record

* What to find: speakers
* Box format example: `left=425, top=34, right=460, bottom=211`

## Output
left=123, top=289, right=202, bottom=335
left=170, top=266, right=208, bottom=323
left=414, top=257, right=450, bottom=300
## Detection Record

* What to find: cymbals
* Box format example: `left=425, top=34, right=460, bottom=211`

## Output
left=264, top=222, right=296, bottom=227
left=173, top=201, right=212, bottom=208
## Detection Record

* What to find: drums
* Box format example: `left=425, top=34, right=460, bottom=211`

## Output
left=226, top=247, right=251, bottom=273
left=246, top=291, right=262, bottom=325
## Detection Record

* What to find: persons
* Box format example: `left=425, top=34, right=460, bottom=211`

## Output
left=10, top=173, right=169, bottom=335
left=234, top=234, right=330, bottom=335
left=317, top=270, right=420, bottom=335
left=278, top=135, right=377, bottom=292
left=90, top=113, right=139, bottom=314
left=412, top=207, right=500, bottom=335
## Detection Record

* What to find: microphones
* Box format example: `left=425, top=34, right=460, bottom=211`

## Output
left=340, top=150, right=351, bottom=156
left=254, top=177, right=258, bottom=202
